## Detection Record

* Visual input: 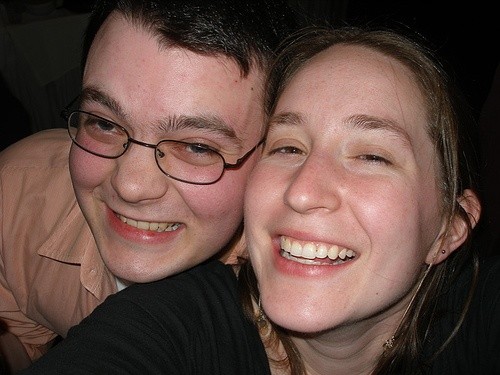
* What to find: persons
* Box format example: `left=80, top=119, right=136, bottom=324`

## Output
left=13, top=17, right=499, bottom=375
left=1, top=1, right=328, bottom=375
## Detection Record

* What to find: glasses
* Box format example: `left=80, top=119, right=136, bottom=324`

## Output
left=67, top=110, right=266, bottom=185
